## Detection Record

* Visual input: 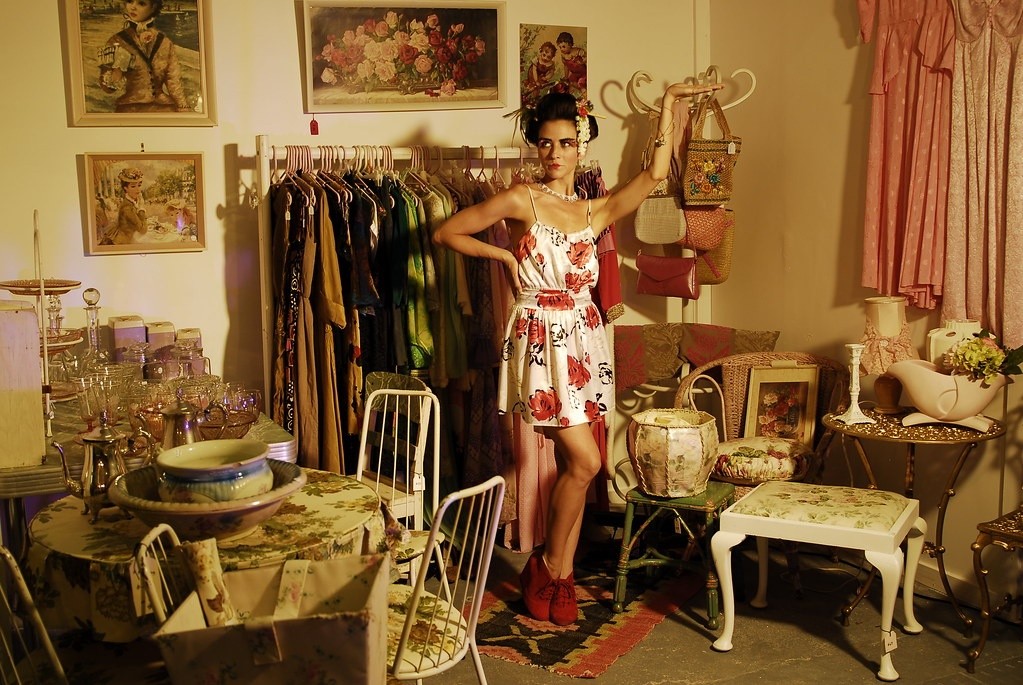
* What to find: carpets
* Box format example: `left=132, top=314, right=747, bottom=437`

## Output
left=462, top=536, right=707, bottom=680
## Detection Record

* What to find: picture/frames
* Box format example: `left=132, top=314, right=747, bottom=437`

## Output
left=64, top=0, right=219, bottom=127
left=744, top=360, right=821, bottom=450
left=84, top=151, right=208, bottom=255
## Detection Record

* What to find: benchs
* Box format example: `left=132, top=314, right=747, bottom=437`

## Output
left=710, top=480, right=923, bottom=682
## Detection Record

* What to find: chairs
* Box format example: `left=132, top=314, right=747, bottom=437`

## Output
left=671, top=351, right=850, bottom=602
left=136, top=524, right=195, bottom=629
left=0, top=545, right=172, bottom=685
left=349, top=372, right=426, bottom=593
left=966, top=505, right=1023, bottom=674
left=354, top=390, right=452, bottom=605
left=387, top=476, right=507, bottom=685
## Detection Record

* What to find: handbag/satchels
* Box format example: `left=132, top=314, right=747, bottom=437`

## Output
left=634, top=195, right=686, bottom=245
left=695, top=208, right=735, bottom=285
left=624, top=138, right=679, bottom=197
left=680, top=94, right=742, bottom=207
left=636, top=253, right=699, bottom=301
left=675, top=206, right=725, bottom=251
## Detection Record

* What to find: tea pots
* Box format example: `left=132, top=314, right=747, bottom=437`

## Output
left=51, top=412, right=156, bottom=524
left=135, top=386, right=228, bottom=465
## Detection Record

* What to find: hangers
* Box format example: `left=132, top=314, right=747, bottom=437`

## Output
left=270, top=144, right=508, bottom=208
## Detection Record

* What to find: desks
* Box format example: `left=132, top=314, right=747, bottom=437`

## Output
left=15, top=465, right=412, bottom=644
left=822, top=404, right=1007, bottom=638
left=0, top=371, right=297, bottom=664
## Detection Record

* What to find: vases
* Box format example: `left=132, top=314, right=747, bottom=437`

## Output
left=926, top=318, right=997, bottom=363
left=303, top=0, right=507, bottom=114
left=888, top=359, right=1015, bottom=433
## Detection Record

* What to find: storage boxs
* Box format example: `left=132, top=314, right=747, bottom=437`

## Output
left=151, top=551, right=391, bottom=685
left=108, top=316, right=205, bottom=379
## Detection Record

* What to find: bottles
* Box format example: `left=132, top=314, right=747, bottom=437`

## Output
left=44, top=288, right=114, bottom=383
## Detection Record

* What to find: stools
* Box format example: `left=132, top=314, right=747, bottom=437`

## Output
left=611, top=481, right=735, bottom=631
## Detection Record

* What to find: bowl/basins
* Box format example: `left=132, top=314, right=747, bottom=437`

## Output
left=106, top=457, right=308, bottom=543
left=154, top=439, right=275, bottom=503
left=126, top=403, right=165, bottom=439
left=196, top=410, right=258, bottom=440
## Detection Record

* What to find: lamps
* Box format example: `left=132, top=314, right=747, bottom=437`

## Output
left=848, top=296, right=921, bottom=414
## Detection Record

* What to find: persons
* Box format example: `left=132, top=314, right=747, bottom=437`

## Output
left=433, top=84, right=725, bottom=625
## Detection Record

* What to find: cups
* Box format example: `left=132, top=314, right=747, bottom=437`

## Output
left=128, top=374, right=262, bottom=431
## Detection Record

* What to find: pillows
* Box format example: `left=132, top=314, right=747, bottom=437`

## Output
left=711, top=437, right=814, bottom=480
left=613, top=325, right=781, bottom=395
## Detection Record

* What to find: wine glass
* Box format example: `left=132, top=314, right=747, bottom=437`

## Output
left=74, top=365, right=140, bottom=445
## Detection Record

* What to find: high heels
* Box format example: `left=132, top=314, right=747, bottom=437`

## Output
left=551, top=571, right=577, bottom=626
left=518, top=551, right=560, bottom=621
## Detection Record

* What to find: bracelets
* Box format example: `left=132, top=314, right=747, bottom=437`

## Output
left=655, top=106, right=674, bottom=148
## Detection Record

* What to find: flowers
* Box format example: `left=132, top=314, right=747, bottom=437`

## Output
left=941, top=329, right=1023, bottom=386
left=576, top=98, right=593, bottom=168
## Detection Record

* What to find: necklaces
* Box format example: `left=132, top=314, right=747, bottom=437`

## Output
left=538, top=181, right=578, bottom=202
